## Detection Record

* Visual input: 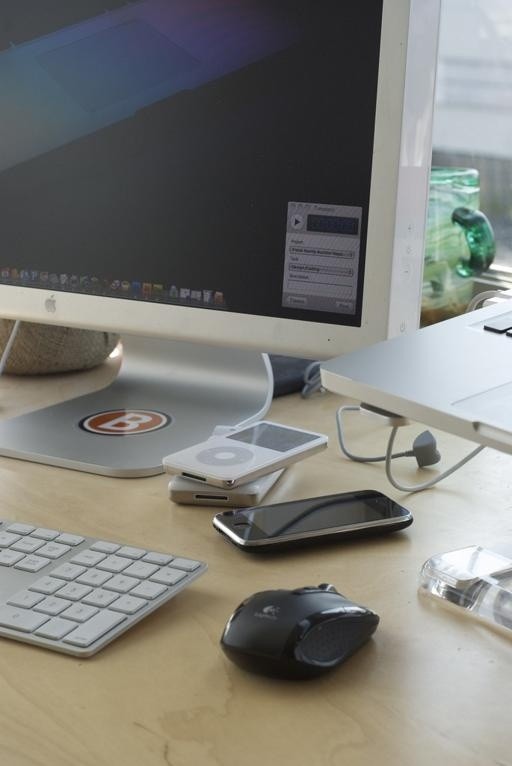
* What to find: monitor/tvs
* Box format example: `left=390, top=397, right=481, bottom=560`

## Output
left=1, top=0, right=440, bottom=479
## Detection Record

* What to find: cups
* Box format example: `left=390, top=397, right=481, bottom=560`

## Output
left=421, top=168, right=495, bottom=320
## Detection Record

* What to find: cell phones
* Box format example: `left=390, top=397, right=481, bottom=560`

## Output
left=211, top=490, right=414, bottom=551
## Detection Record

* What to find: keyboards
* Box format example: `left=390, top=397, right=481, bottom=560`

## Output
left=0, top=520, right=207, bottom=658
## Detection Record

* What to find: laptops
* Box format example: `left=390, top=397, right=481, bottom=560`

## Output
left=318, top=299, right=510, bottom=458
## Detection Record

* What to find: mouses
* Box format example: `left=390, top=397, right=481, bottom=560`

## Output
left=220, top=582, right=377, bottom=678
